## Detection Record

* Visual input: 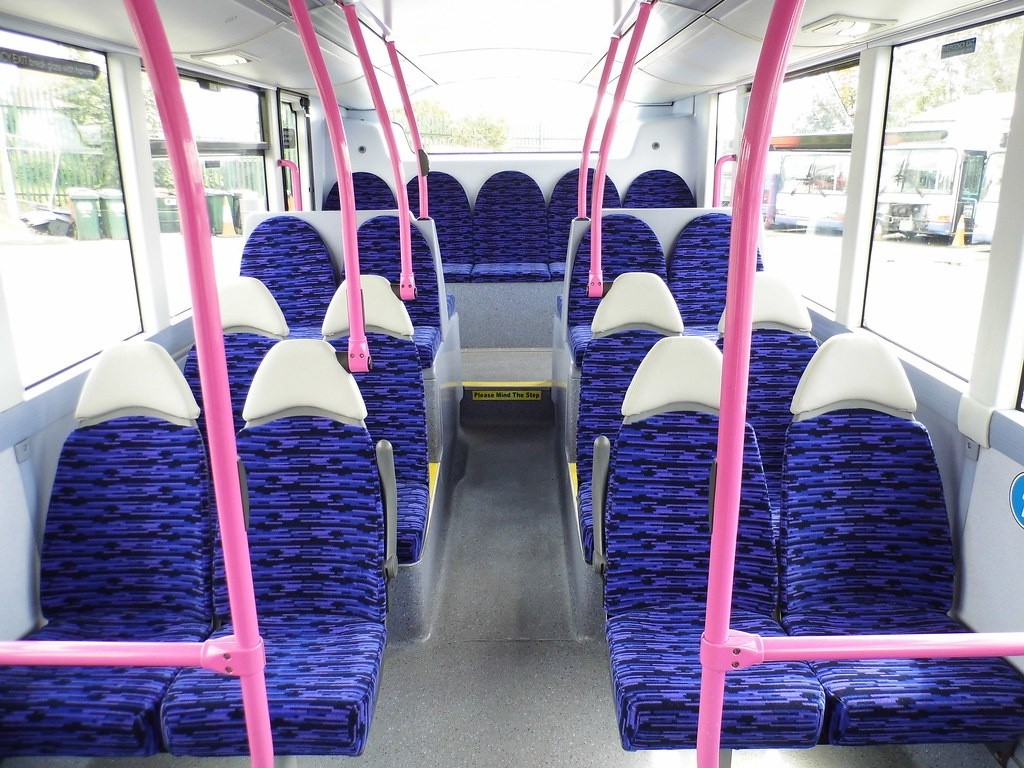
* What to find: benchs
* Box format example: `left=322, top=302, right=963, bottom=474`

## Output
left=0, top=162, right=1024, bottom=768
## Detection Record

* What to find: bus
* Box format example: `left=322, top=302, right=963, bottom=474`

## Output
left=763, top=142, right=1007, bottom=246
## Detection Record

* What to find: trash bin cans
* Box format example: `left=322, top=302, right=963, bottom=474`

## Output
left=66, top=188, right=102, bottom=240
left=205, top=189, right=234, bottom=235
left=720, top=170, right=734, bottom=206
left=98, top=189, right=129, bottom=240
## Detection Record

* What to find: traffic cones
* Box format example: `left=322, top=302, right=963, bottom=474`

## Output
left=946, top=213, right=968, bottom=247
